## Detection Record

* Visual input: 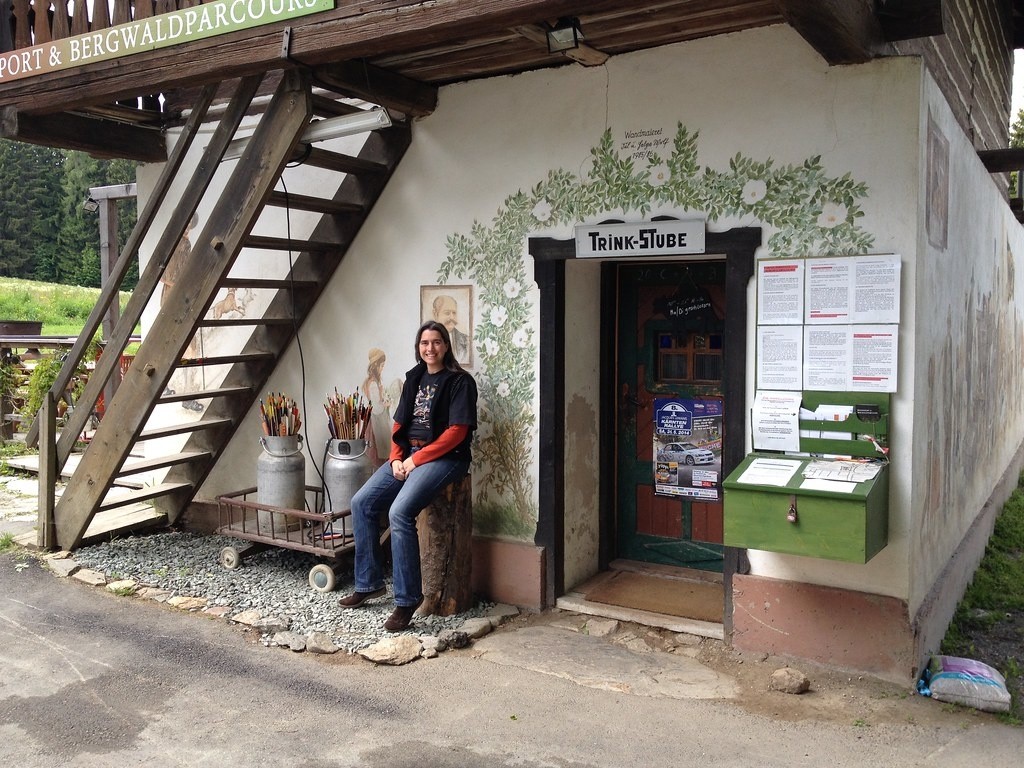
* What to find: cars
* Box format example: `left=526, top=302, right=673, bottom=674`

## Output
left=657, top=441, right=716, bottom=467
left=657, top=463, right=677, bottom=483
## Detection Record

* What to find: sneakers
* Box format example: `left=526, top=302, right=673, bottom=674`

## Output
left=338, top=587, right=386, bottom=609
left=384, top=594, right=424, bottom=632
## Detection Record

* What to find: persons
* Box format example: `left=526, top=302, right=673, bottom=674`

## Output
left=337, top=321, right=478, bottom=632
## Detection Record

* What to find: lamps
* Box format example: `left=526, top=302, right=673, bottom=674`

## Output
left=546, top=15, right=583, bottom=54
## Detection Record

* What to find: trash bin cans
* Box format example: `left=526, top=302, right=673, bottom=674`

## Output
left=96, top=349, right=136, bottom=420
